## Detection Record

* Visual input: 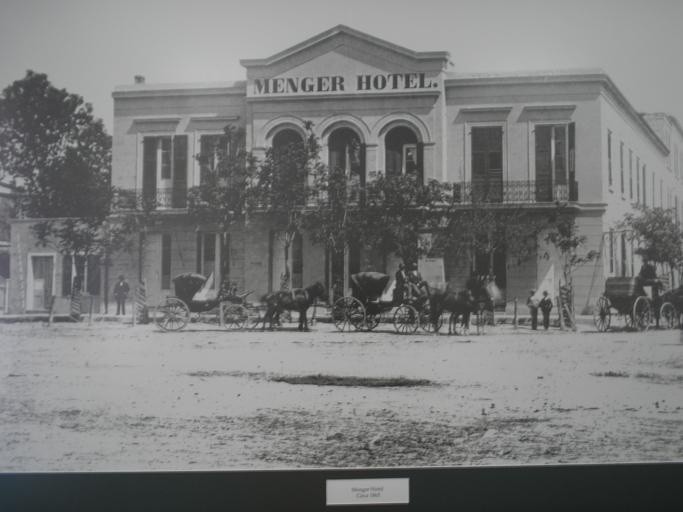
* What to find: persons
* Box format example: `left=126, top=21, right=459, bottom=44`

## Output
left=526, top=288, right=540, bottom=331
left=331, top=277, right=343, bottom=308
left=395, top=263, right=422, bottom=305
left=111, top=273, right=130, bottom=314
left=639, top=257, right=657, bottom=280
left=539, top=291, right=554, bottom=329
left=409, top=263, right=434, bottom=296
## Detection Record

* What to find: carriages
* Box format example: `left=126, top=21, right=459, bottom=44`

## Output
left=153, top=273, right=325, bottom=332
left=330, top=271, right=503, bottom=335
left=593, top=277, right=682, bottom=333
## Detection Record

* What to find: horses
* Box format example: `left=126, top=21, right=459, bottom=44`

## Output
left=430, top=275, right=491, bottom=335
left=260, top=280, right=329, bottom=331
